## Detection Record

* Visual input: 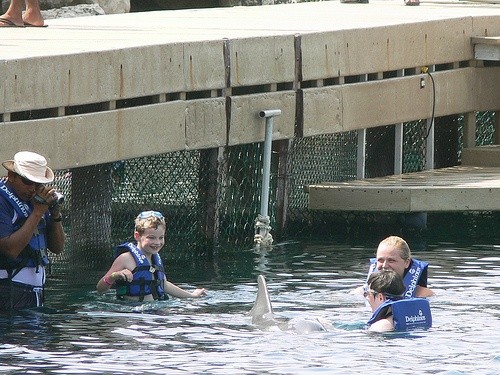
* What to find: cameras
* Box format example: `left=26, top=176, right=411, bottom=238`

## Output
left=51, top=192, right=65, bottom=205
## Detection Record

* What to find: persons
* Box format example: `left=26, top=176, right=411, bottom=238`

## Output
left=95, top=209, right=208, bottom=302
left=364, top=269, right=433, bottom=331
left=0, top=0, right=48, bottom=28
left=352, top=235, right=435, bottom=297
left=0, top=150, right=65, bottom=312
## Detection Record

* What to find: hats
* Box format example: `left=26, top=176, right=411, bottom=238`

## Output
left=3, top=151, right=54, bottom=183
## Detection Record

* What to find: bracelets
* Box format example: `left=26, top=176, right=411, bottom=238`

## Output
left=111, top=271, right=116, bottom=280
left=52, top=213, right=62, bottom=222
left=104, top=276, right=110, bottom=287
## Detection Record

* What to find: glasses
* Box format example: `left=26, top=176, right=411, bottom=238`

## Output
left=14, top=176, right=42, bottom=186
left=136, top=212, right=163, bottom=232
left=363, top=292, right=378, bottom=298
